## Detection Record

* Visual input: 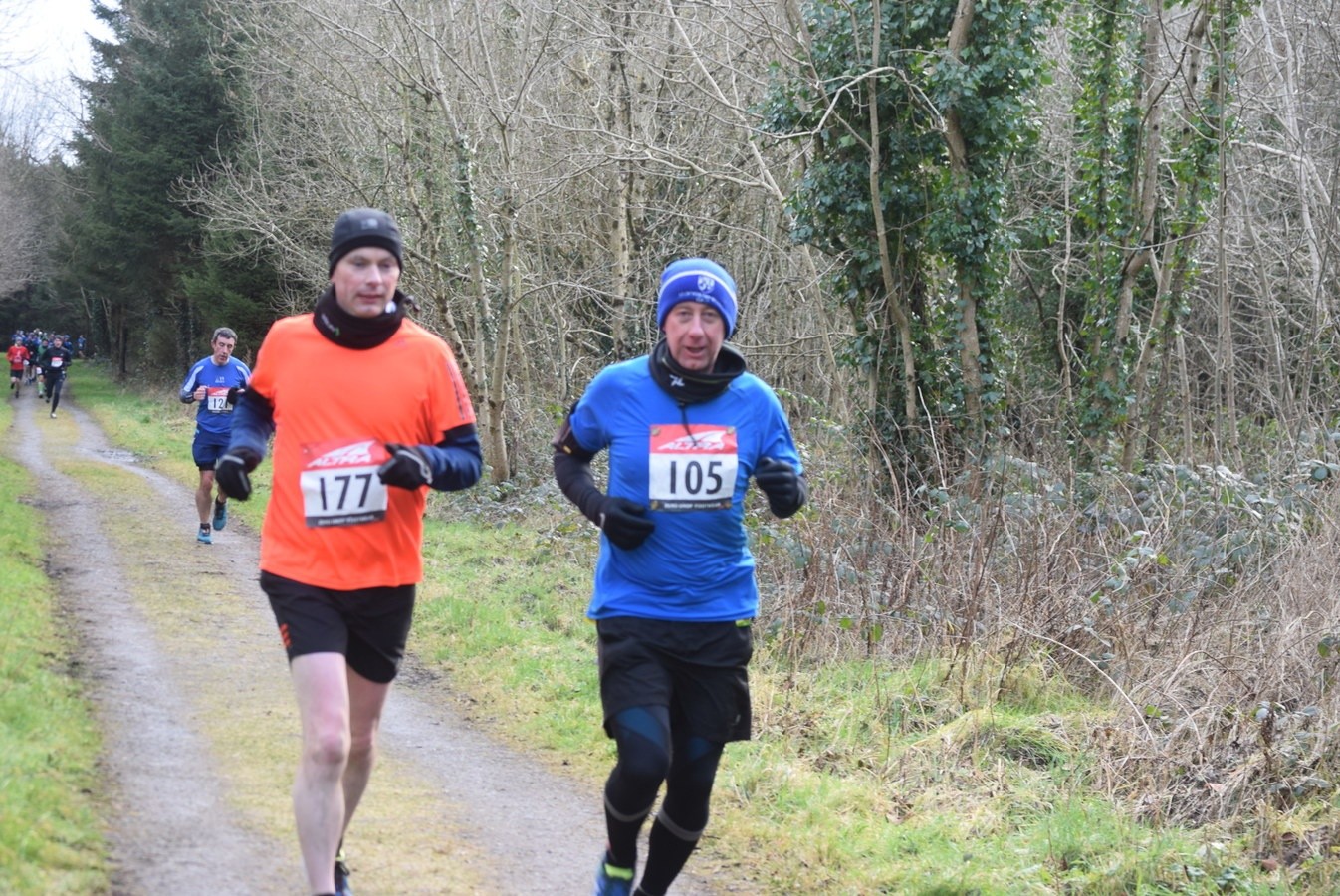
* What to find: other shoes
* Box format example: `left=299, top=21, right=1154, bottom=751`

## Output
left=11, top=377, right=57, bottom=419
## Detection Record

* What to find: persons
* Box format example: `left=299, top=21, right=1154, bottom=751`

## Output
left=550, top=257, right=808, bottom=895
left=11, top=327, right=74, bottom=399
left=6, top=337, right=30, bottom=399
left=77, top=334, right=97, bottom=362
left=214, top=206, right=485, bottom=895
left=179, top=327, right=252, bottom=546
left=40, top=335, right=72, bottom=419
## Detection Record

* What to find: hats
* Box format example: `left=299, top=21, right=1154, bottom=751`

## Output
left=655, top=257, right=738, bottom=341
left=327, top=206, right=403, bottom=277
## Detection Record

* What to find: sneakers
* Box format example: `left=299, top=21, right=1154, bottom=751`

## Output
left=212, top=496, right=228, bottom=530
left=197, top=525, right=212, bottom=545
left=333, top=847, right=352, bottom=896
left=595, top=849, right=636, bottom=896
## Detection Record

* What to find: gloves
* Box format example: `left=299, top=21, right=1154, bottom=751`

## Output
left=753, top=457, right=796, bottom=504
left=216, top=450, right=260, bottom=501
left=378, top=441, right=433, bottom=490
left=599, top=500, right=654, bottom=549
